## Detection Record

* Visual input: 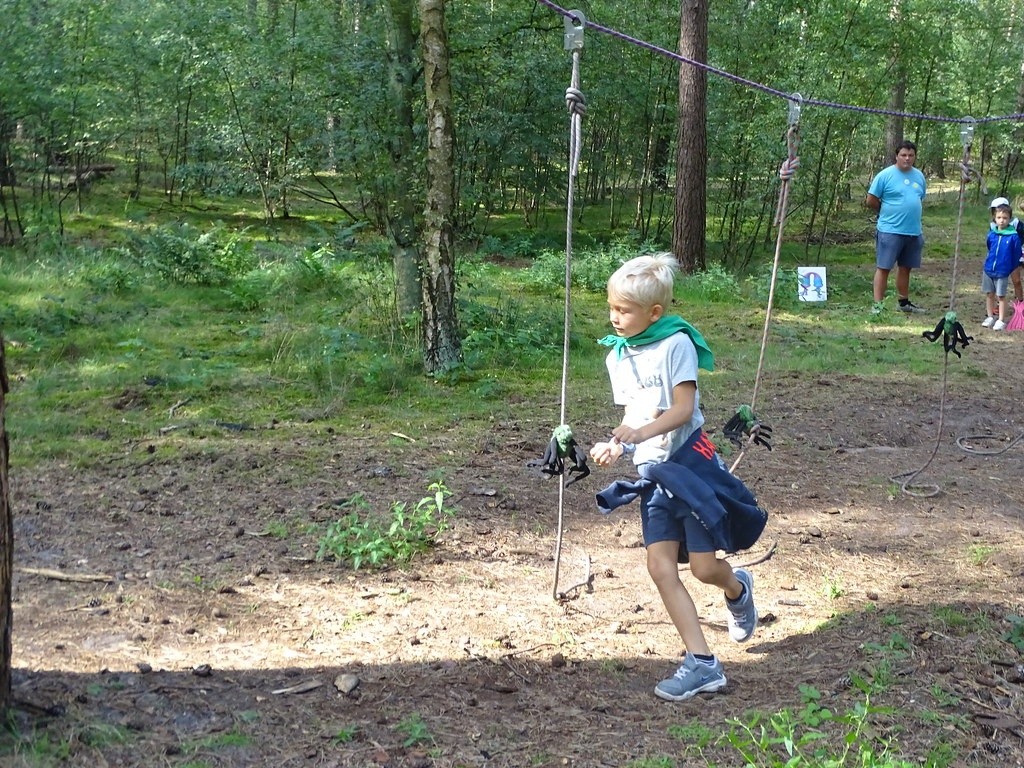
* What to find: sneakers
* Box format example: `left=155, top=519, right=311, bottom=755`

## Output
left=723, top=568, right=759, bottom=644
left=654, top=650, right=727, bottom=701
left=981, top=316, right=994, bottom=328
left=992, top=320, right=1007, bottom=331
left=896, top=301, right=929, bottom=313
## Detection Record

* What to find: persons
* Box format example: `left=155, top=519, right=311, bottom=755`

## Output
left=866, top=140, right=927, bottom=314
left=589, top=254, right=768, bottom=702
left=981, top=197, right=1024, bottom=331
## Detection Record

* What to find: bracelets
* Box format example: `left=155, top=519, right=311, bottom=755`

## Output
left=619, top=444, right=627, bottom=457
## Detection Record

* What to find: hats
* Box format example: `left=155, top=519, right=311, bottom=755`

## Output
left=987, top=196, right=1010, bottom=209
